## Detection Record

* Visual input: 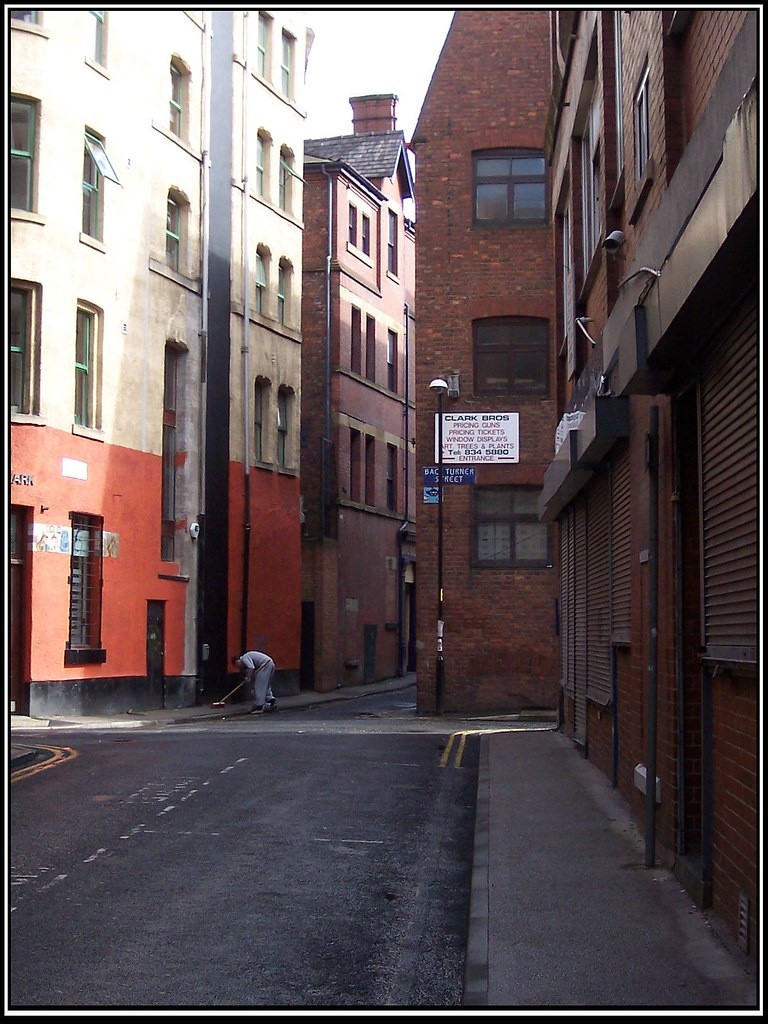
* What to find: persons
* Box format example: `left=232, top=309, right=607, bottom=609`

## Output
left=231, top=650, right=279, bottom=714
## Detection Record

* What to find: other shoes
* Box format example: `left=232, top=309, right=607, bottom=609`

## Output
left=252, top=709, right=263, bottom=714
left=268, top=704, right=278, bottom=711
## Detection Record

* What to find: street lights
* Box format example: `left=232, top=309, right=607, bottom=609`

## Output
left=428, top=379, right=448, bottom=717
